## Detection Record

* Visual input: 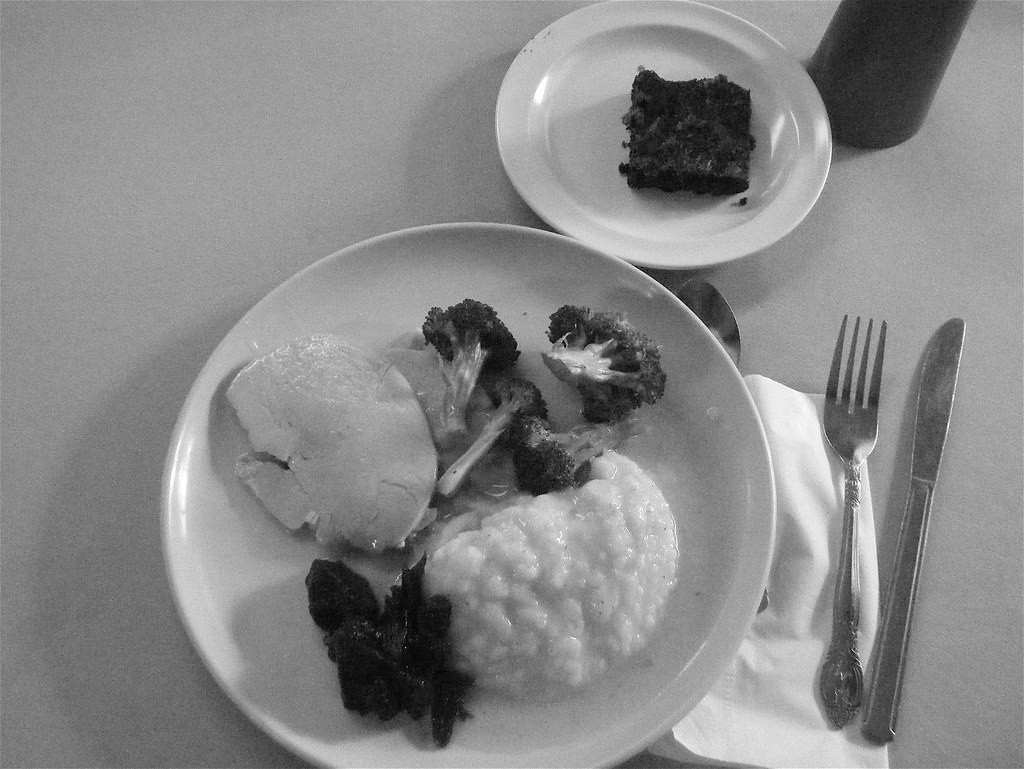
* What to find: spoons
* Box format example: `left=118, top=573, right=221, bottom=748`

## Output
left=676, top=275, right=770, bottom=614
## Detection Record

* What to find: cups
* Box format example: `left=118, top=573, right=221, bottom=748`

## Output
left=805, top=0, right=977, bottom=150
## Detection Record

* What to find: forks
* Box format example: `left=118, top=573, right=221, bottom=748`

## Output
left=819, top=315, right=888, bottom=731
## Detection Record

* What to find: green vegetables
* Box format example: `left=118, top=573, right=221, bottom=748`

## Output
left=420, top=299, right=668, bottom=497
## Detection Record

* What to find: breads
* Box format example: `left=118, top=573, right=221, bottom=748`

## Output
left=616, top=69, right=754, bottom=195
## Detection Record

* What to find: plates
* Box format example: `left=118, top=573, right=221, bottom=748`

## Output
left=494, top=0, right=832, bottom=270
left=159, top=222, right=777, bottom=769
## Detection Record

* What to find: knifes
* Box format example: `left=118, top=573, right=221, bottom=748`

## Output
left=858, top=318, right=965, bottom=746
